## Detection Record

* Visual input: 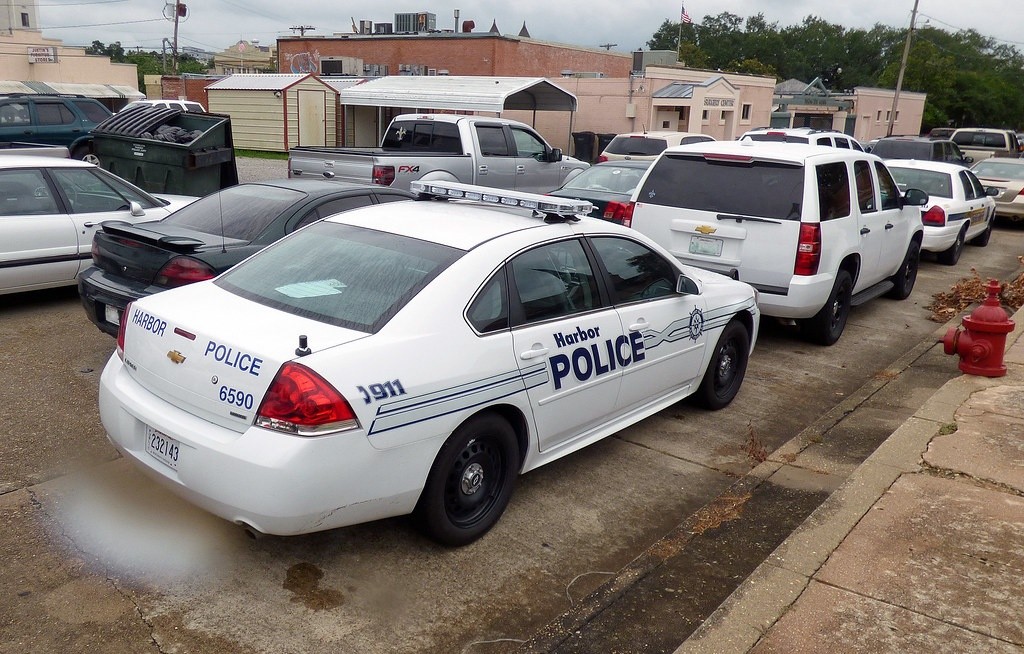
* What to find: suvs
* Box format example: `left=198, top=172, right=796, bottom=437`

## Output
left=595, top=129, right=718, bottom=164
left=737, top=126, right=867, bottom=153
left=0, top=92, right=117, bottom=161
left=622, top=140, right=930, bottom=346
left=868, top=134, right=975, bottom=169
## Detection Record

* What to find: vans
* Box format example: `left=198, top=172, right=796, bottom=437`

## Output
left=119, top=99, right=208, bottom=113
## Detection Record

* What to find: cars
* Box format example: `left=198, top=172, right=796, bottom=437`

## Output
left=966, top=158, right=1024, bottom=223
left=98, top=180, right=762, bottom=548
left=546, top=160, right=657, bottom=230
left=0, top=154, right=208, bottom=298
left=926, top=128, right=956, bottom=141
left=77, top=176, right=419, bottom=341
left=878, top=158, right=999, bottom=267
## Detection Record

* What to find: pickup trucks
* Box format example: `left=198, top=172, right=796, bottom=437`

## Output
left=287, top=114, right=593, bottom=196
left=946, top=126, right=1024, bottom=167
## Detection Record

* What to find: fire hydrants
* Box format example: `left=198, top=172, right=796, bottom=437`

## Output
left=938, top=279, right=1016, bottom=378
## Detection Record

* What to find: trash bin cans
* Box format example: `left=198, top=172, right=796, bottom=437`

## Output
left=88, top=104, right=239, bottom=197
left=594, top=133, right=617, bottom=157
left=571, top=131, right=595, bottom=163
